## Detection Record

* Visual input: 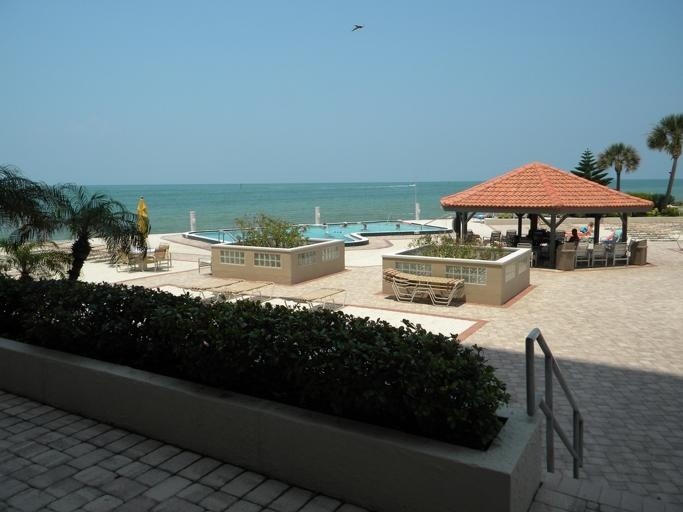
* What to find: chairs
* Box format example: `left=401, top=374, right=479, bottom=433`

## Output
left=590, top=243, right=607, bottom=268
left=574, top=242, right=589, bottom=268
left=382, top=267, right=466, bottom=308
left=481, top=231, right=564, bottom=266
left=116, top=244, right=171, bottom=273
left=611, top=241, right=629, bottom=266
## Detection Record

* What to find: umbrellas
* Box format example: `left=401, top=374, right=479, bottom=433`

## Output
left=135, top=196, right=150, bottom=247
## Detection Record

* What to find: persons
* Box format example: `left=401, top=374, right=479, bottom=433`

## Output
left=607, top=227, right=615, bottom=241
left=586, top=221, right=593, bottom=236
left=569, top=229, right=579, bottom=242
